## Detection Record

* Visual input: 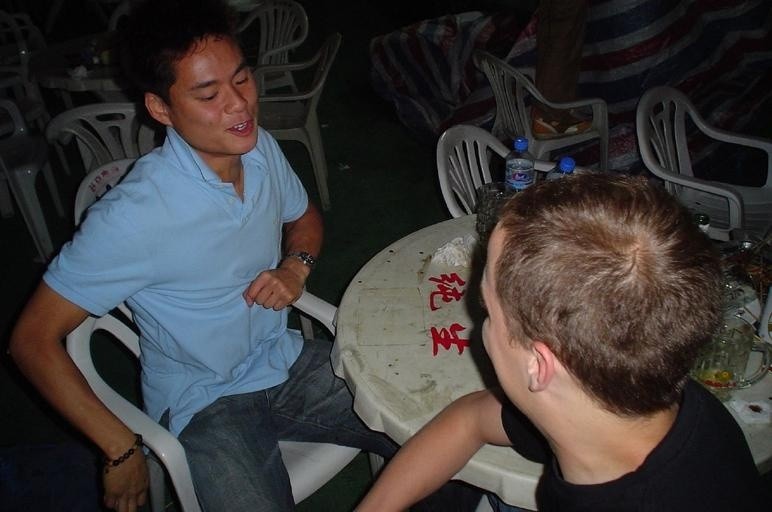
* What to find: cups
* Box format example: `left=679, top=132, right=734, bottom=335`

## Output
left=690, top=314, right=772, bottom=403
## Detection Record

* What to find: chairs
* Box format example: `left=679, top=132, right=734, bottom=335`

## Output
left=633, top=82, right=772, bottom=244
left=2, top=3, right=346, bottom=266
left=470, top=46, right=613, bottom=173
left=63, top=289, right=385, bottom=511
left=436, top=124, right=593, bottom=219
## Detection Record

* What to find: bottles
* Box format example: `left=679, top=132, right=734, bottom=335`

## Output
left=505, top=138, right=536, bottom=194
left=545, top=157, right=577, bottom=183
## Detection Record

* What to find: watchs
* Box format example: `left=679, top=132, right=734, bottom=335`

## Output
left=287, top=251, right=316, bottom=268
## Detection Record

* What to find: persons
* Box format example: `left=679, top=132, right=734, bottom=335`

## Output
left=10, top=0, right=400, bottom=512
left=355, top=171, right=772, bottom=512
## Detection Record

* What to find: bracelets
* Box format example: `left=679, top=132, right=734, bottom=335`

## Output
left=108, top=433, right=143, bottom=466
